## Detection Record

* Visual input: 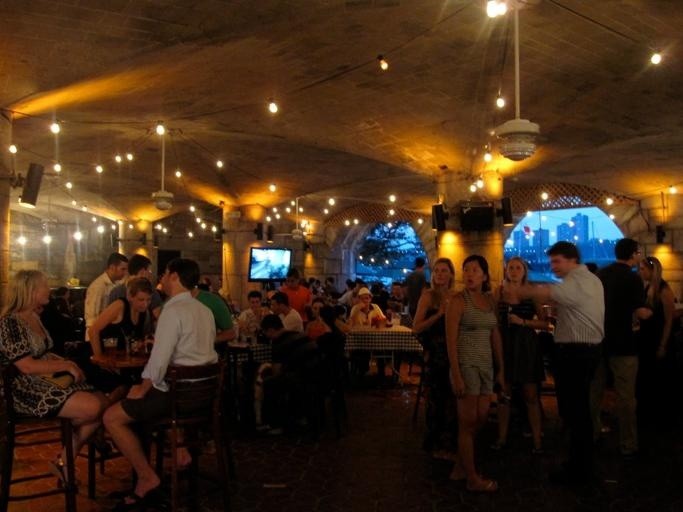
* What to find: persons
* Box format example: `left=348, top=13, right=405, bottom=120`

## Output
left=0, top=269, right=127, bottom=493
left=338, top=257, right=422, bottom=386
left=102, top=260, right=218, bottom=509
left=445, top=255, right=511, bottom=495
left=413, top=259, right=461, bottom=463
left=492, top=256, right=553, bottom=465
left=83, top=254, right=237, bottom=387
left=596, top=238, right=646, bottom=463
left=501, top=240, right=606, bottom=487
left=54, top=288, right=86, bottom=331
left=636, top=257, right=675, bottom=441
left=230, top=267, right=347, bottom=432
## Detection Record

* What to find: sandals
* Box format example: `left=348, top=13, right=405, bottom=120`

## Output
left=48, top=454, right=79, bottom=489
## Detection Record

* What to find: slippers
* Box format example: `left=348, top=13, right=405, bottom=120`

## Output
left=467, top=480, right=502, bottom=494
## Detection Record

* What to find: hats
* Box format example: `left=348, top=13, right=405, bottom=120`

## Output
left=358, top=288, right=372, bottom=296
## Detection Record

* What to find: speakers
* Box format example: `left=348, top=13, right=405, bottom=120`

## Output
left=459, top=206, right=494, bottom=230
left=657, top=225, right=665, bottom=244
left=267, top=225, right=274, bottom=244
left=500, top=197, right=514, bottom=226
left=432, top=205, right=446, bottom=231
left=257, top=223, right=263, bottom=241
left=18, top=163, right=44, bottom=208
left=143, top=234, right=147, bottom=244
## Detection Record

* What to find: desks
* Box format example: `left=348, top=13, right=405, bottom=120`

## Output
left=229, top=323, right=424, bottom=380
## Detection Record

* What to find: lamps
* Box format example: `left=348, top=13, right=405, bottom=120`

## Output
left=12, top=160, right=43, bottom=207
left=495, top=1, right=541, bottom=164
left=151, top=131, right=175, bottom=209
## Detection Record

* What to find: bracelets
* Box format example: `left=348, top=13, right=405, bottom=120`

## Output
left=522, top=318, right=527, bottom=327
left=438, top=312, right=442, bottom=318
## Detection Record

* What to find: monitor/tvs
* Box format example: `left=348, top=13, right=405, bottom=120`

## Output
left=249, top=246, right=292, bottom=282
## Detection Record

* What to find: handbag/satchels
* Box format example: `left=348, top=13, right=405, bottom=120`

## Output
left=36, top=352, right=79, bottom=390
